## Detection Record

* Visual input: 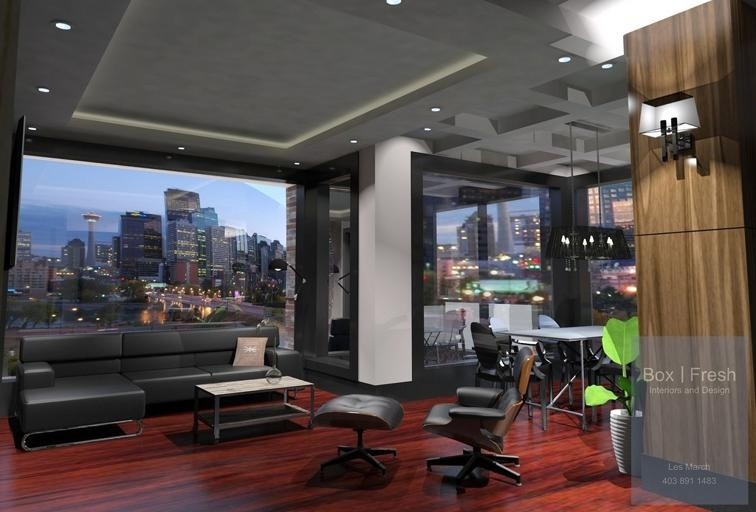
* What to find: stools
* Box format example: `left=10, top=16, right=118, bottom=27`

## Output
left=312, top=393, right=405, bottom=476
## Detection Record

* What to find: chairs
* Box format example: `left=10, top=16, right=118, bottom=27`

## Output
left=422, top=304, right=601, bottom=494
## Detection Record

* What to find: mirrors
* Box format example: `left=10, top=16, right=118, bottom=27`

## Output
left=326, top=178, right=352, bottom=361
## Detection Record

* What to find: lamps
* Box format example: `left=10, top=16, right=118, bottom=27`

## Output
left=268, top=258, right=308, bottom=285
left=638, top=89, right=702, bottom=163
left=545, top=121, right=631, bottom=272
left=328, top=264, right=351, bottom=295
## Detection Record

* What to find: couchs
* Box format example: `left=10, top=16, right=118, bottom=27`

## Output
left=13, top=325, right=302, bottom=452
left=328, top=317, right=351, bottom=353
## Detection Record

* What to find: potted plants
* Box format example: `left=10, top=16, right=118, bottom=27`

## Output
left=585, top=314, right=644, bottom=476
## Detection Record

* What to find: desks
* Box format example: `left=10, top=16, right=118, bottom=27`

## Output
left=495, top=324, right=605, bottom=433
